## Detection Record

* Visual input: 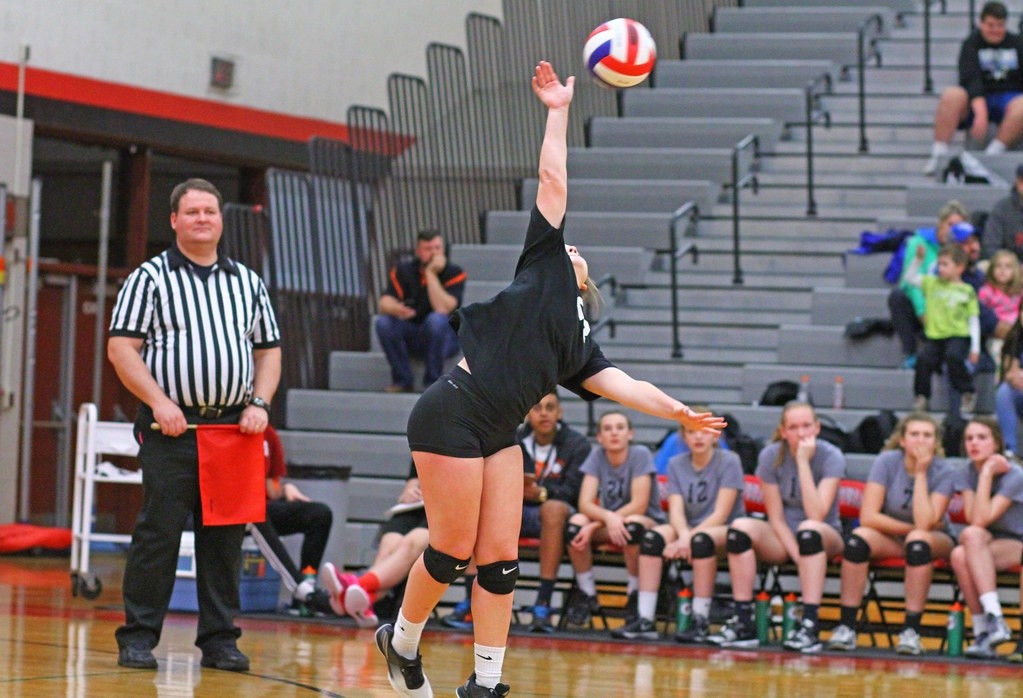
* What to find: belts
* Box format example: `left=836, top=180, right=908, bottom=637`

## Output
left=177, top=403, right=247, bottom=419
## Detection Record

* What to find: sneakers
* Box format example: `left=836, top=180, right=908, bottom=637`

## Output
left=984, top=612, right=1011, bottom=647
left=200, top=633, right=250, bottom=672
left=441, top=598, right=473, bottom=630
left=965, top=631, right=999, bottom=660
left=527, top=604, right=553, bottom=634
left=675, top=617, right=710, bottom=643
left=611, top=617, right=660, bottom=641
left=826, top=619, right=858, bottom=651
left=623, top=590, right=638, bottom=626
left=705, top=614, right=760, bottom=648
left=896, top=626, right=921, bottom=656
left=374, top=623, right=434, bottom=698
left=566, top=594, right=599, bottom=628
left=456, top=671, right=510, bottom=698
left=783, top=618, right=821, bottom=651
left=117, top=643, right=158, bottom=669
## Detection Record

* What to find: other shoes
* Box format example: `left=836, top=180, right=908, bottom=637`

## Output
left=1007, top=641, right=1023, bottom=663
left=1002, top=449, right=1023, bottom=465
left=913, top=394, right=930, bottom=412
left=383, top=383, right=414, bottom=393
left=297, top=587, right=334, bottom=618
left=344, top=577, right=379, bottom=630
left=323, top=561, right=357, bottom=616
left=958, top=391, right=978, bottom=413
left=926, top=153, right=943, bottom=176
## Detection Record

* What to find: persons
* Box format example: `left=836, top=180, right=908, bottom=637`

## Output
left=196, top=423, right=268, bottom=526
left=923, top=0, right=1023, bottom=174
left=242, top=166, right=1023, bottom=664
left=706, top=399, right=847, bottom=652
left=562, top=411, right=669, bottom=634
left=374, top=60, right=727, bottom=698
left=829, top=415, right=959, bottom=657
left=377, top=228, right=468, bottom=392
left=107, top=177, right=283, bottom=670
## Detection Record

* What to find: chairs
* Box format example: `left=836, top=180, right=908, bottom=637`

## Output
left=517, top=456, right=1023, bottom=655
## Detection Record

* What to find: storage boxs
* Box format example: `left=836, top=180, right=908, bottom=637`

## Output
left=168, top=528, right=281, bottom=612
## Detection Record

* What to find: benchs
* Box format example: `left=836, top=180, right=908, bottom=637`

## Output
left=274, top=0, right=1023, bottom=574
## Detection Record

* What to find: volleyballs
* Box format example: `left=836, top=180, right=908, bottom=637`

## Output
left=581, top=18, right=657, bottom=88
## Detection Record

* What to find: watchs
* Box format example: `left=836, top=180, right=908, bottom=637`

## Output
left=249, top=396, right=271, bottom=412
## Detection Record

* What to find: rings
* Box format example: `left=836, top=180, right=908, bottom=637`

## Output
left=254, top=423, right=262, bottom=429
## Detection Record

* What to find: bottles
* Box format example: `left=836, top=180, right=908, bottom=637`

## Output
left=946, top=601, right=965, bottom=658
left=754, top=591, right=771, bottom=645
left=675, top=588, right=693, bottom=635
left=832, top=377, right=844, bottom=409
left=796, top=375, right=810, bottom=404
left=300, top=565, right=317, bottom=618
left=782, top=593, right=798, bottom=641
